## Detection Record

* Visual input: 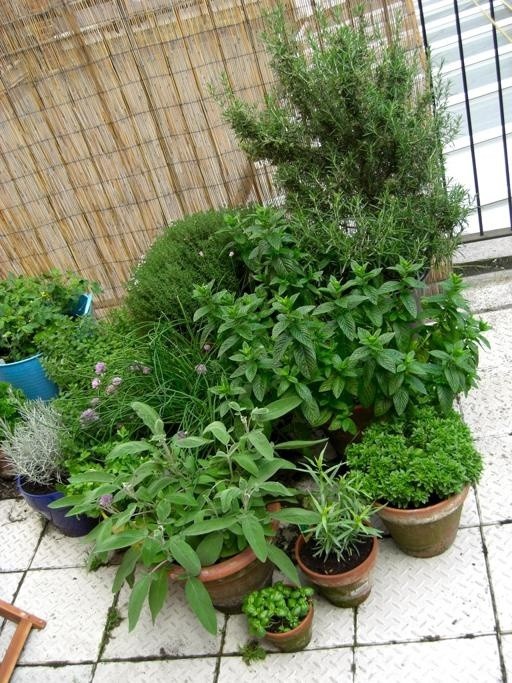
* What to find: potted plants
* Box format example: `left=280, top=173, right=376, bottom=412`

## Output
left=48, top=401, right=320, bottom=639
left=295, top=442, right=383, bottom=611
left=0, top=267, right=105, bottom=400
left=242, top=581, right=315, bottom=653
left=1, top=394, right=104, bottom=540
left=346, top=408, right=485, bottom=559
